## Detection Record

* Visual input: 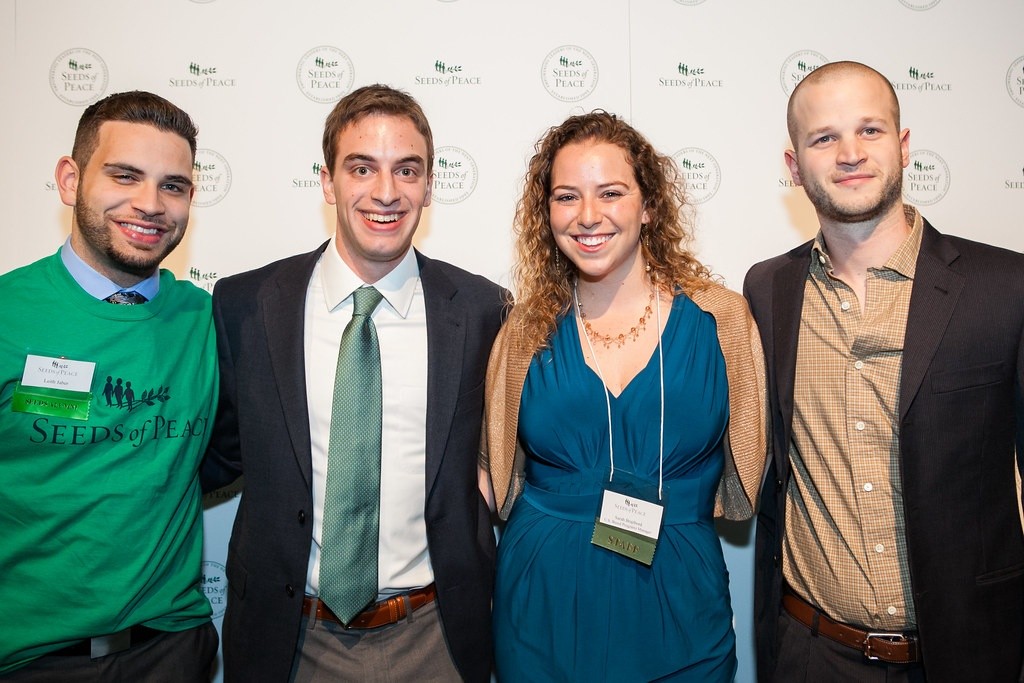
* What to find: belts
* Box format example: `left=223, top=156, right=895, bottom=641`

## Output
left=302, top=583, right=435, bottom=630
left=780, top=592, right=919, bottom=664
left=50, top=625, right=162, bottom=657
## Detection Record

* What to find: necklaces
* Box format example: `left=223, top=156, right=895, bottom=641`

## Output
left=574, top=273, right=654, bottom=349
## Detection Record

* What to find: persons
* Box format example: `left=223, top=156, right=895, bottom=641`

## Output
left=480, top=109, right=770, bottom=683
left=744, top=61, right=1024, bottom=683
left=197, top=83, right=514, bottom=683
left=0, top=89, right=218, bottom=683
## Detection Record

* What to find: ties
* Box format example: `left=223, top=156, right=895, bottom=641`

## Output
left=106, top=290, right=148, bottom=305
left=319, top=284, right=384, bottom=627
